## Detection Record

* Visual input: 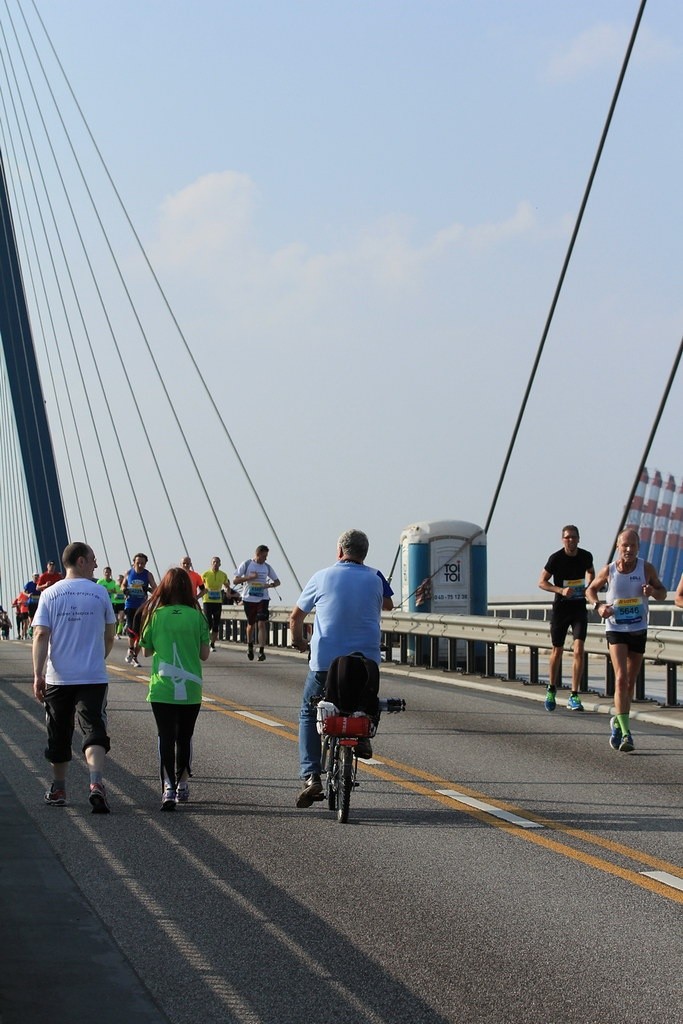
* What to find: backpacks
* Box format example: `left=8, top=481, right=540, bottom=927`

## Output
left=324, top=650, right=380, bottom=716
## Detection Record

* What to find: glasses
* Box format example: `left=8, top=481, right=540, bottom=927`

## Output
left=564, top=535, right=577, bottom=540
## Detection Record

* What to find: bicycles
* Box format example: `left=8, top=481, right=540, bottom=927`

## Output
left=291, top=642, right=391, bottom=823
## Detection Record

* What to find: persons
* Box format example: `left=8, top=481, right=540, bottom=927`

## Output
left=585, top=529, right=667, bottom=752
left=28, top=541, right=118, bottom=815
left=201, top=558, right=231, bottom=652
left=290, top=527, right=395, bottom=808
left=674, top=572, right=683, bottom=608
left=233, top=544, right=281, bottom=661
left=35, top=560, right=63, bottom=592
left=181, top=556, right=206, bottom=600
left=132, top=567, right=212, bottom=812
left=24, top=573, right=41, bottom=625
left=93, top=566, right=128, bottom=639
left=121, top=553, right=157, bottom=667
left=537, top=525, right=595, bottom=712
left=221, top=579, right=244, bottom=605
left=0, top=612, right=12, bottom=641
left=11, top=591, right=33, bottom=640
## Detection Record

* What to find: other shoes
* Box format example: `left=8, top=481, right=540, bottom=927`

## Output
left=357, top=738, right=373, bottom=760
left=125, top=649, right=141, bottom=667
left=296, top=773, right=324, bottom=808
left=247, top=644, right=255, bottom=662
left=257, top=654, right=265, bottom=661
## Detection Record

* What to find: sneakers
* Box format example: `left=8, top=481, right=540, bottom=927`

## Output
left=609, top=717, right=622, bottom=750
left=177, top=788, right=188, bottom=804
left=544, top=685, right=556, bottom=712
left=88, top=783, right=110, bottom=813
left=160, top=790, right=176, bottom=812
left=43, top=783, right=65, bottom=807
left=566, top=696, right=584, bottom=711
left=620, top=731, right=634, bottom=753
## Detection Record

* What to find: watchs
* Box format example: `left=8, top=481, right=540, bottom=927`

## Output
left=593, top=601, right=601, bottom=609
